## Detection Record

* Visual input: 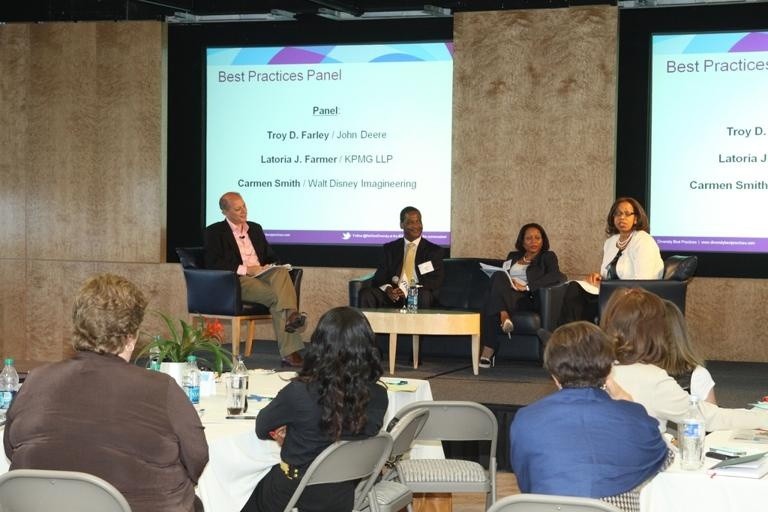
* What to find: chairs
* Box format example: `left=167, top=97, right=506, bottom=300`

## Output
left=284, top=431, right=394, bottom=512
left=0, top=469, right=132, bottom=512
left=486, top=494, right=622, bottom=512
left=354, top=408, right=430, bottom=512
left=385, top=401, right=498, bottom=512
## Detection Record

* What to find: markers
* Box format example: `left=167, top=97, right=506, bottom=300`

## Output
left=705, top=451, right=739, bottom=461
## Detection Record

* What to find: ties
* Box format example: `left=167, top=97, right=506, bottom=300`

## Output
left=403, top=242, right=414, bottom=284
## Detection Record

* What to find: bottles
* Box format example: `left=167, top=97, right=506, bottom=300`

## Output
left=151, top=363, right=160, bottom=372
left=678, top=395, right=706, bottom=471
left=231, top=355, right=249, bottom=413
left=0, top=358, right=20, bottom=420
left=181, top=355, right=201, bottom=411
left=407, top=279, right=418, bottom=310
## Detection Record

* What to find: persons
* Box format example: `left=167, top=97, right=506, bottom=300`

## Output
left=479, top=223, right=560, bottom=368
left=359, top=207, right=444, bottom=367
left=538, top=197, right=664, bottom=342
left=509, top=321, right=674, bottom=512
left=202, top=191, right=307, bottom=367
left=601, top=286, right=768, bottom=437
left=664, top=299, right=717, bottom=406
left=241, top=306, right=389, bottom=512
left=3, top=274, right=209, bottom=512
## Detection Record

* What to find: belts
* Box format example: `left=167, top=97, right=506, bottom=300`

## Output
left=279, top=459, right=300, bottom=480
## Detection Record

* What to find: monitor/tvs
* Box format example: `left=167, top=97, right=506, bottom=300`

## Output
left=615, top=1, right=768, bottom=280
left=163, top=15, right=453, bottom=270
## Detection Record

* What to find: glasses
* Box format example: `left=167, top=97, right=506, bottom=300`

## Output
left=612, top=211, right=637, bottom=216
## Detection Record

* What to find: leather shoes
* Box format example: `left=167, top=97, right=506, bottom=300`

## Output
left=281, top=352, right=305, bottom=367
left=285, top=310, right=308, bottom=333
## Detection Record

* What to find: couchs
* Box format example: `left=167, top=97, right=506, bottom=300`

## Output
left=597, top=254, right=697, bottom=327
left=174, top=246, right=303, bottom=366
left=349, top=258, right=572, bottom=367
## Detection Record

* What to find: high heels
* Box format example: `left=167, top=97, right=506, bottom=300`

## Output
left=478, top=351, right=495, bottom=368
left=500, top=318, right=514, bottom=340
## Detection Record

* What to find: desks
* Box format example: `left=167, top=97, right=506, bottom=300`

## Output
left=638, top=429, right=768, bottom=512
left=0, top=371, right=445, bottom=512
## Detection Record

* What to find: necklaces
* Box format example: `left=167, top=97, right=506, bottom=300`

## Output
left=617, top=232, right=634, bottom=247
left=523, top=256, right=533, bottom=264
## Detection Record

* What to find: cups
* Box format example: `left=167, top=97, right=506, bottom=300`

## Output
left=225, top=375, right=246, bottom=417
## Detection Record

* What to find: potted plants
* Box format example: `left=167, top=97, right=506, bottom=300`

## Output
left=134, top=308, right=235, bottom=386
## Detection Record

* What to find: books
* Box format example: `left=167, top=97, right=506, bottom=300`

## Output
left=707, top=450, right=768, bottom=480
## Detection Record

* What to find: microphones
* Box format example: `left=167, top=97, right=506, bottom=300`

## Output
left=392, top=275, right=400, bottom=289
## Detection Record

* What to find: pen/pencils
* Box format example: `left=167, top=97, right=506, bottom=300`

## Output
left=225, top=415, right=255, bottom=420
left=385, top=379, right=408, bottom=385
